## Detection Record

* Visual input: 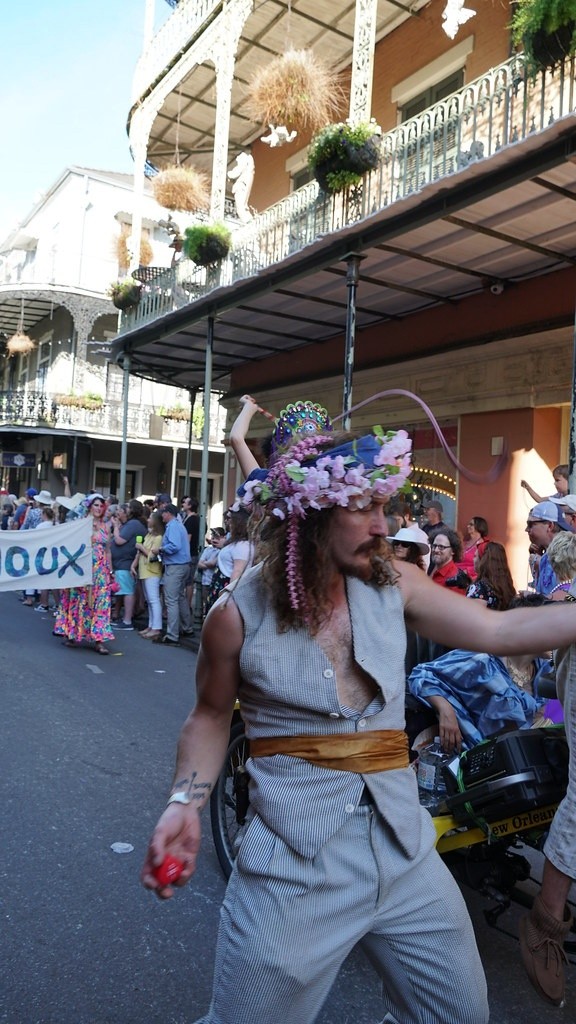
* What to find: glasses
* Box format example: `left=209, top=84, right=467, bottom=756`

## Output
left=468, top=522, right=475, bottom=526
left=392, top=539, right=412, bottom=548
left=431, top=544, right=452, bottom=550
left=527, top=519, right=551, bottom=529
left=225, top=515, right=233, bottom=521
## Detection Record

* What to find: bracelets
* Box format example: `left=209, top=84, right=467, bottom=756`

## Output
left=167, top=793, right=202, bottom=816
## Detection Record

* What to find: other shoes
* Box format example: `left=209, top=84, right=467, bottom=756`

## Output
left=519, top=891, right=573, bottom=1006
left=18, top=595, right=61, bottom=613
left=109, top=610, right=194, bottom=645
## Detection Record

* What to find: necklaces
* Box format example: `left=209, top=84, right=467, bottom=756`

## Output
left=464, top=536, right=482, bottom=554
left=548, top=577, right=575, bottom=595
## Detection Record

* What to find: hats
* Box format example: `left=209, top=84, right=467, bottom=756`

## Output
left=530, top=500, right=574, bottom=531
left=421, top=501, right=443, bottom=512
left=56, top=493, right=86, bottom=512
left=159, top=504, right=178, bottom=516
left=384, top=526, right=430, bottom=556
left=33, top=491, right=54, bottom=505
left=548, top=494, right=576, bottom=511
left=153, top=494, right=171, bottom=504
left=27, top=489, right=39, bottom=498
left=13, top=497, right=27, bottom=508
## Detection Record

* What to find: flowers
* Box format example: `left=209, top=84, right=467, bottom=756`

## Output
left=307, top=115, right=382, bottom=192
left=242, top=425, right=413, bottom=519
left=104, top=280, right=140, bottom=297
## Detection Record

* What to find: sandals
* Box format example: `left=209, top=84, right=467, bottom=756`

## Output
left=66, top=638, right=75, bottom=647
left=95, top=644, right=109, bottom=655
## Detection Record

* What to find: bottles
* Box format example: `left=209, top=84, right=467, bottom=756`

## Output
left=418, top=736, right=454, bottom=808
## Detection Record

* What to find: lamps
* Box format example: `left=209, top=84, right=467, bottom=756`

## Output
left=37, top=450, right=48, bottom=480
left=158, top=461, right=168, bottom=492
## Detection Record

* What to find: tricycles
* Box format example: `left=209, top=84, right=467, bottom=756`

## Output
left=211, top=696, right=569, bottom=930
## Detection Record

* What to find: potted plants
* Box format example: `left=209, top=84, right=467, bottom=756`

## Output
left=55, top=391, right=107, bottom=413
left=184, top=221, right=230, bottom=267
left=503, top=0, right=576, bottom=79
left=150, top=405, right=205, bottom=440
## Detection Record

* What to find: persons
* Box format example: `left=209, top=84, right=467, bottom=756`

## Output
left=0, top=486, right=207, bottom=654
left=261, top=124, right=297, bottom=148
left=228, top=151, right=255, bottom=225
left=159, top=220, right=180, bottom=237
left=140, top=394, right=576, bottom=1024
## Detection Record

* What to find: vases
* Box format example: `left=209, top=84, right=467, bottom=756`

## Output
left=314, top=136, right=383, bottom=196
left=112, top=287, right=140, bottom=310
